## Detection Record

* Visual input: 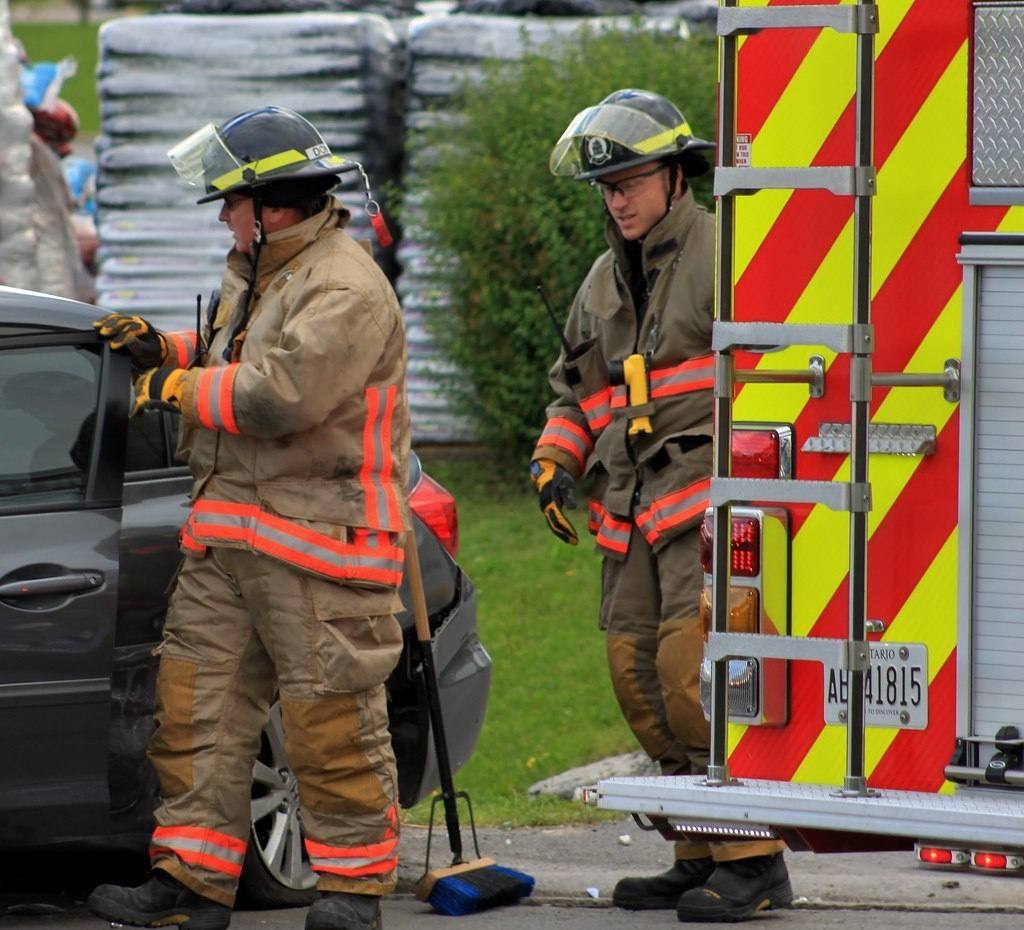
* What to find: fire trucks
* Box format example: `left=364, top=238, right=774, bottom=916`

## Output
left=575, top=0, right=1024, bottom=877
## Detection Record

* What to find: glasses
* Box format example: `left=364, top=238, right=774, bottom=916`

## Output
left=595, top=162, right=671, bottom=199
left=224, top=196, right=249, bottom=210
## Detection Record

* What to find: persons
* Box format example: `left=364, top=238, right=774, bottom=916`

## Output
left=13, top=36, right=101, bottom=303
left=92, top=104, right=409, bottom=930
left=530, top=87, right=792, bottom=923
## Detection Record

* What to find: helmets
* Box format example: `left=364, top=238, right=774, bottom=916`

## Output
left=27, top=98, right=78, bottom=160
left=196, top=106, right=359, bottom=204
left=573, top=89, right=717, bottom=181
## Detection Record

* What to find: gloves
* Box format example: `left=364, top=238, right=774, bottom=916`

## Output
left=530, top=458, right=579, bottom=546
left=91, top=314, right=167, bottom=374
left=127, top=366, right=189, bottom=420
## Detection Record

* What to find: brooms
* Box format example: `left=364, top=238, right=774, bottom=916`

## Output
left=405, top=509, right=535, bottom=917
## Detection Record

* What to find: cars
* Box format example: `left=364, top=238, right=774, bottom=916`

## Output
left=0, top=281, right=493, bottom=914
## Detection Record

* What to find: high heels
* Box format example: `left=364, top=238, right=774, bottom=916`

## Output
left=677, top=851, right=793, bottom=922
left=612, top=855, right=716, bottom=910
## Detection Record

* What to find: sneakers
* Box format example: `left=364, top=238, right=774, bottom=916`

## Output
left=305, top=892, right=382, bottom=930
left=88, top=868, right=231, bottom=930
